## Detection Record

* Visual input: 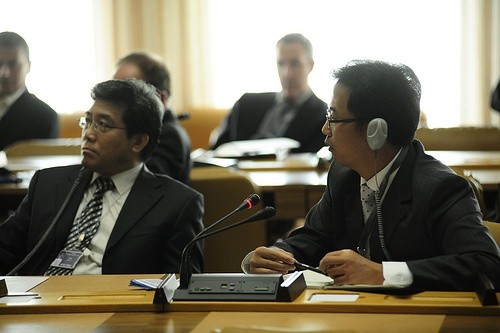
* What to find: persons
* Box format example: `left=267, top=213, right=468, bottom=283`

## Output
left=241, top=61, right=500, bottom=293
left=0, top=32, right=59, bottom=149
left=113, top=53, right=191, bottom=185
left=207, top=32, right=328, bottom=155
left=0, top=79, right=205, bottom=290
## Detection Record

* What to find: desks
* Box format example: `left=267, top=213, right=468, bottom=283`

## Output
left=0, top=148, right=500, bottom=216
left=0, top=243, right=500, bottom=333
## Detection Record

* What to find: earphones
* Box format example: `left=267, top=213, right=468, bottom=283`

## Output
left=366, top=118, right=388, bottom=150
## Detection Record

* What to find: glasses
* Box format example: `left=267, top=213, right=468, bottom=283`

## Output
left=78, top=117, right=130, bottom=133
left=325, top=109, right=363, bottom=129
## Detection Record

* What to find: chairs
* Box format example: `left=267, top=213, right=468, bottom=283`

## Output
left=189, top=168, right=267, bottom=271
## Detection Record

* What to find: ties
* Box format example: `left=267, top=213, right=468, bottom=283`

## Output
left=41, top=176, right=116, bottom=276
left=262, top=98, right=294, bottom=137
left=361, top=185, right=375, bottom=226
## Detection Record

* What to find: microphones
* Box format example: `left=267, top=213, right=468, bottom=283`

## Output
left=176, top=113, right=190, bottom=121
left=172, top=193, right=284, bottom=301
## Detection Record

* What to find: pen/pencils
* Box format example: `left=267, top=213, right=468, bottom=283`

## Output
left=283, top=261, right=329, bottom=277
